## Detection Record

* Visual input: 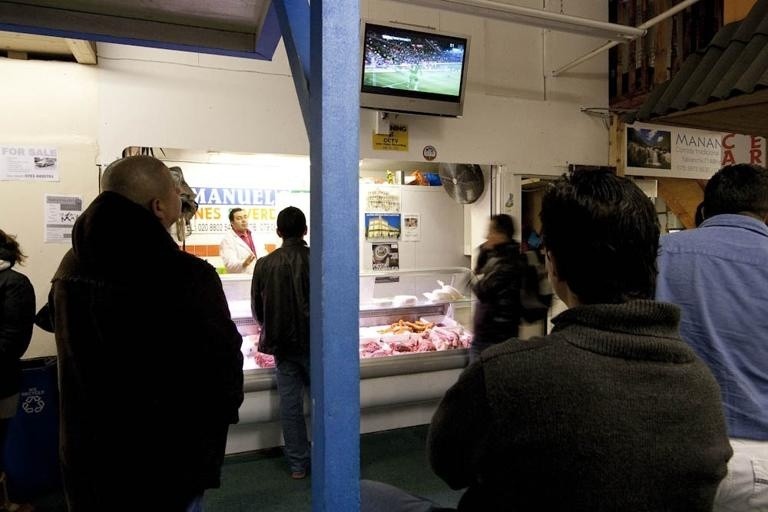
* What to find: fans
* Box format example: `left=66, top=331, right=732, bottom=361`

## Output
left=438, top=163, right=485, bottom=203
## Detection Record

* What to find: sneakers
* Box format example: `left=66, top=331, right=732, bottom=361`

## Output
left=292, top=464, right=311, bottom=478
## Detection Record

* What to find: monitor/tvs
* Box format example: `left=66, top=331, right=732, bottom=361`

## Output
left=359, top=16, right=473, bottom=117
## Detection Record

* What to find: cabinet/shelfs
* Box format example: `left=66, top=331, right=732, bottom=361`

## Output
left=219, top=268, right=478, bottom=456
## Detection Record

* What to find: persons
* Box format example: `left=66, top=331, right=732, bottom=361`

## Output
left=652, top=164, right=768, bottom=511
left=1, top=227, right=38, bottom=508
left=247, top=205, right=311, bottom=481
left=45, top=154, right=248, bottom=512
left=358, top=159, right=735, bottom=508
left=456, top=212, right=526, bottom=365
left=217, top=207, right=270, bottom=274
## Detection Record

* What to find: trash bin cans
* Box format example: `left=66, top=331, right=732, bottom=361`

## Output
left=2, top=356, right=59, bottom=504
